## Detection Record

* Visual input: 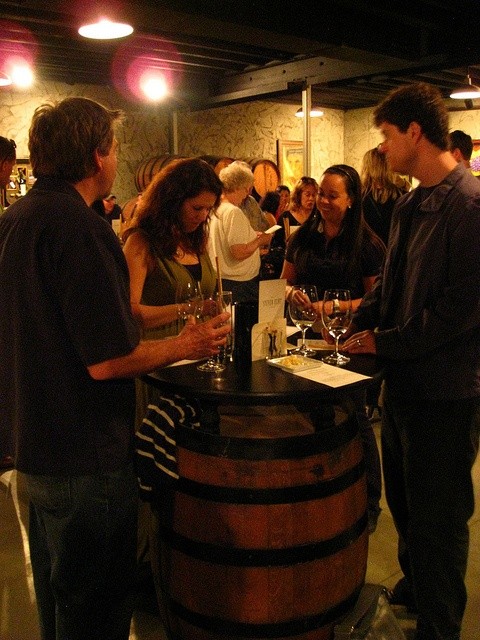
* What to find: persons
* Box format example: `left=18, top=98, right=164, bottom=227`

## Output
left=280, top=164, right=387, bottom=534
left=0, top=95, right=231, bottom=640
left=258, top=192, right=279, bottom=227
left=322, top=83, right=479, bottom=640
left=278, top=186, right=293, bottom=214
left=359, top=149, right=410, bottom=245
left=122, top=158, right=225, bottom=607
left=231, top=161, right=273, bottom=283
left=269, top=176, right=320, bottom=278
left=449, top=130, right=473, bottom=176
left=210, top=159, right=273, bottom=364
left=0, top=135, right=17, bottom=188
left=103, top=193, right=125, bottom=225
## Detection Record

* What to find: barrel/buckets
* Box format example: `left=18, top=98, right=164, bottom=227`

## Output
left=168, top=406, right=370, bottom=640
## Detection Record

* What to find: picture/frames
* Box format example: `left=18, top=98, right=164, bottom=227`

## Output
left=277, top=140, right=305, bottom=188
left=470, top=140, right=480, bottom=175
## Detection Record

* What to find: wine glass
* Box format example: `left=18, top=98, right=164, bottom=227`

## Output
left=321, top=289, right=352, bottom=369
left=191, top=299, right=226, bottom=373
left=177, top=281, right=201, bottom=328
left=288, top=283, right=319, bottom=358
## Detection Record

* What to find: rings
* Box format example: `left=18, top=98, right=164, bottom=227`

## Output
left=357, top=340, right=362, bottom=347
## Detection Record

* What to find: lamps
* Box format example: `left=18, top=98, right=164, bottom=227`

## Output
left=296, top=105, right=323, bottom=119
left=448, top=63, right=480, bottom=100
left=78, top=17, right=135, bottom=40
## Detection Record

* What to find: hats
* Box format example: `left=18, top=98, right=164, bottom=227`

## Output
left=105, top=193, right=117, bottom=200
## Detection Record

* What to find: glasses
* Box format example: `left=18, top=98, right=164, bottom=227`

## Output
left=1, top=138, right=17, bottom=169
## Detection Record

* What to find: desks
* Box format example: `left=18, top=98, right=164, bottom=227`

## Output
left=135, top=331, right=388, bottom=406
